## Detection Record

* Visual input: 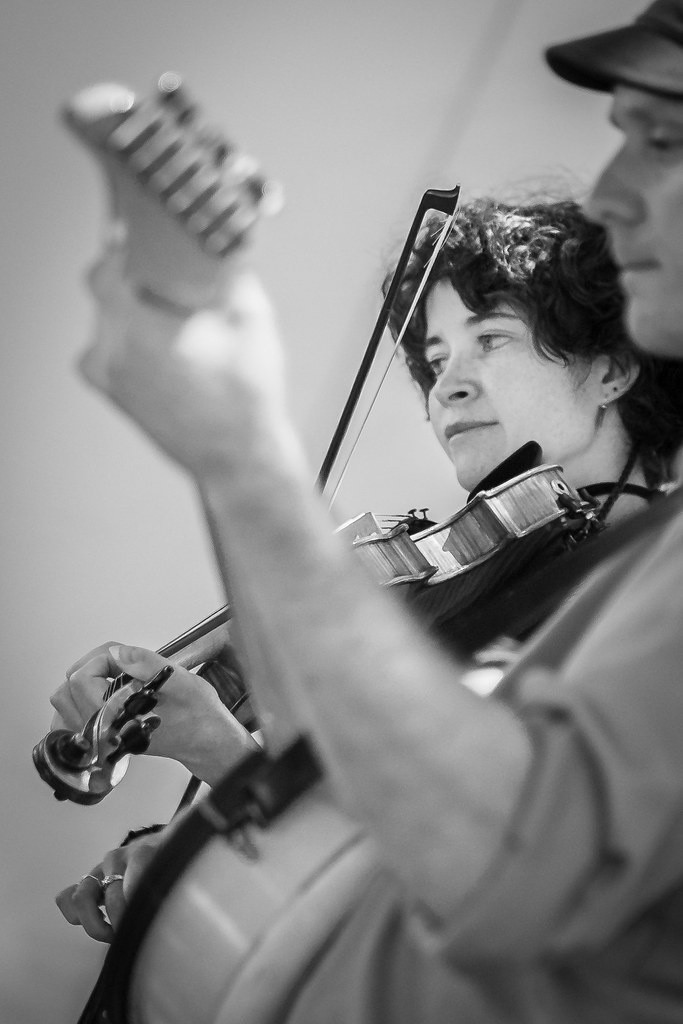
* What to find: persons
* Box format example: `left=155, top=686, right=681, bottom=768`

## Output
left=48, top=0, right=683, bottom=1024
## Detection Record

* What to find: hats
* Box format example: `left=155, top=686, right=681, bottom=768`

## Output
left=546, top=0, right=683, bottom=98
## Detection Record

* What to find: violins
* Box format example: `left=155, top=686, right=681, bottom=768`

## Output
left=31, top=464, right=606, bottom=806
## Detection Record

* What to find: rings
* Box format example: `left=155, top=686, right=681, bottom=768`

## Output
left=82, top=875, right=98, bottom=881
left=101, top=874, right=123, bottom=886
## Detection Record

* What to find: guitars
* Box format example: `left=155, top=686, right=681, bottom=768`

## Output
left=57, top=67, right=517, bottom=1024
